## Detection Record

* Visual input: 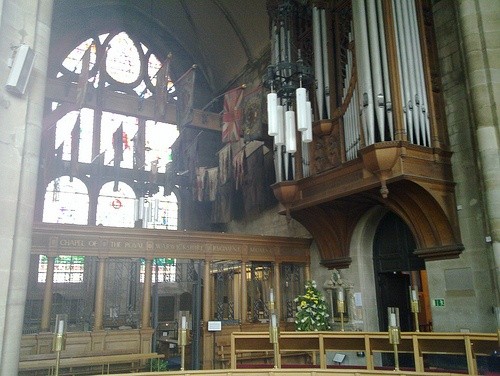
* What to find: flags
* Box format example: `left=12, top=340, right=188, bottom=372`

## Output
left=244, top=86, right=265, bottom=142
left=175, top=68, right=195, bottom=130
left=73, top=46, right=91, bottom=106
left=222, top=87, right=245, bottom=142
left=94, top=52, right=107, bottom=107
left=47, top=113, right=271, bottom=226
left=154, top=57, right=168, bottom=125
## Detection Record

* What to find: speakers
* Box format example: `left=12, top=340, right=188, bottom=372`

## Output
left=6, top=44, right=38, bottom=96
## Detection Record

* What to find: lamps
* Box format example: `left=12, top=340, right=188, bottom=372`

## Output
left=52, top=313, right=67, bottom=376
left=410, top=285, right=421, bottom=331
left=388, top=307, right=402, bottom=371
left=6, top=44, right=36, bottom=96
left=337, top=286, right=346, bottom=332
left=262, top=49, right=315, bottom=154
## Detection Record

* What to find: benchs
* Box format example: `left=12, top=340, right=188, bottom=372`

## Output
left=214, top=322, right=324, bottom=371
left=15, top=328, right=164, bottom=376
left=155, top=338, right=193, bottom=366
